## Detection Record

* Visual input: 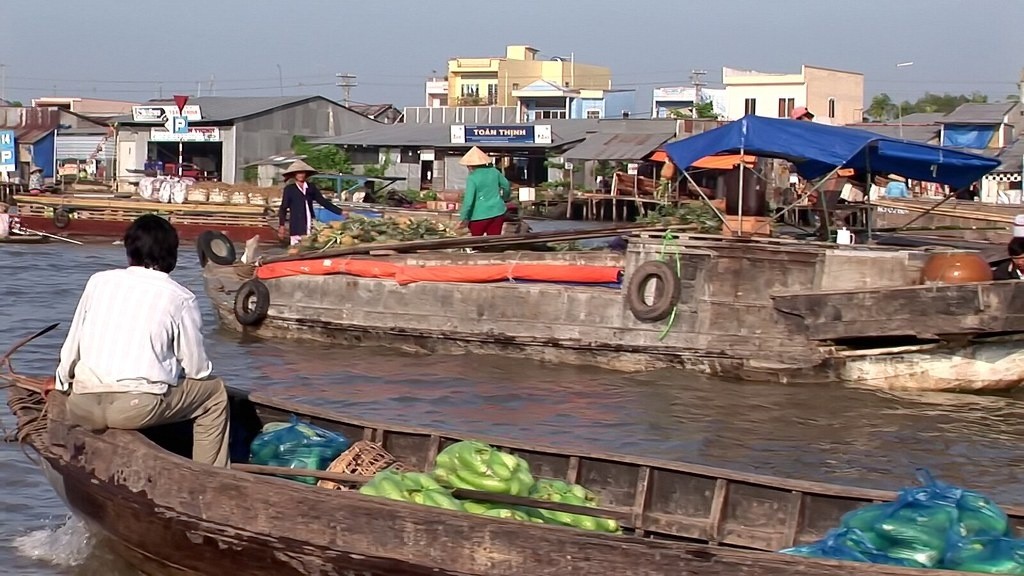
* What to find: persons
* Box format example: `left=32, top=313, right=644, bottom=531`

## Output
left=993, top=237, right=1024, bottom=279
left=54, top=214, right=230, bottom=470
left=28, top=166, right=45, bottom=194
left=156, top=157, right=163, bottom=177
left=789, top=106, right=849, bottom=240
left=458, top=146, right=511, bottom=236
left=885, top=173, right=909, bottom=198
left=278, top=159, right=349, bottom=246
left=611, top=162, right=625, bottom=185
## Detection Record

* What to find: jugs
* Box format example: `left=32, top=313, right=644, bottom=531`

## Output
left=836, top=226, right=855, bottom=244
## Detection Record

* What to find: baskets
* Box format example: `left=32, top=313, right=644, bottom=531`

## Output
left=317, top=440, right=422, bottom=492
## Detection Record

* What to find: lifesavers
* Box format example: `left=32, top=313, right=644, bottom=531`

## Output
left=626, top=260, right=681, bottom=323
left=198, top=229, right=236, bottom=267
left=264, top=207, right=276, bottom=218
left=54, top=211, right=70, bottom=228
left=232, top=279, right=270, bottom=326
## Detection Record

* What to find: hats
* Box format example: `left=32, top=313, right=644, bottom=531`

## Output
left=282, top=159, right=318, bottom=178
left=888, top=173, right=906, bottom=182
left=30, top=166, right=43, bottom=174
left=458, top=146, right=493, bottom=165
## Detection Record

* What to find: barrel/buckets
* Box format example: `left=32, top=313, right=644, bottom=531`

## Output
left=726, top=169, right=765, bottom=217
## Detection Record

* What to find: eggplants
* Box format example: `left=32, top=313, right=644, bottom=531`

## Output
left=358, top=443, right=624, bottom=536
left=252, top=422, right=350, bottom=485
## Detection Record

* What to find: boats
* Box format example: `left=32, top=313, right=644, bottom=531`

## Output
left=869, top=193, right=1024, bottom=250
left=196, top=114, right=1023, bottom=397
left=8, top=195, right=290, bottom=244
left=0, top=320, right=1023, bottom=576
left=615, top=172, right=713, bottom=200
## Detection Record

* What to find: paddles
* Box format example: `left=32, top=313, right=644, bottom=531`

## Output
left=230, top=459, right=560, bottom=512
left=21, top=227, right=83, bottom=245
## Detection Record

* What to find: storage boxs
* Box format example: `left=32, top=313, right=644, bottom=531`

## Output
left=723, top=216, right=770, bottom=243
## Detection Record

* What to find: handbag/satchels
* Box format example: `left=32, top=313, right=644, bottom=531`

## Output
left=462, top=499, right=546, bottom=525
left=359, top=470, right=468, bottom=512
left=774, top=468, right=1024, bottom=575
left=529, top=478, right=622, bottom=534
left=247, top=413, right=349, bottom=485
left=431, top=441, right=535, bottom=497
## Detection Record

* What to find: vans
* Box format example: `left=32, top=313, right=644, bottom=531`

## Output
left=164, top=163, right=205, bottom=181
